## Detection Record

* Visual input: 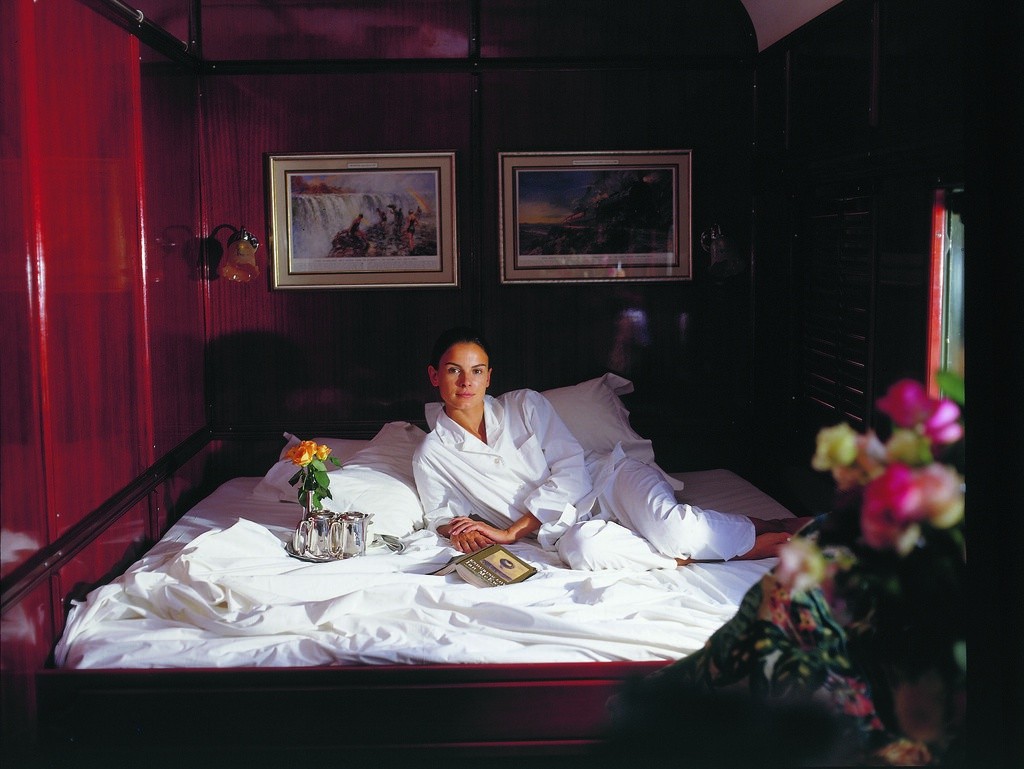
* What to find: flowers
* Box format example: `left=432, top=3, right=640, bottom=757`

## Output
left=776, top=380, right=964, bottom=598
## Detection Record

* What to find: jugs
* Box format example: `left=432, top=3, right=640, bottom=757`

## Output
left=292, top=508, right=342, bottom=560
left=325, top=511, right=376, bottom=560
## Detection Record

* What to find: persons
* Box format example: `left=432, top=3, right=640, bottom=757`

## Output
left=413, top=324, right=819, bottom=574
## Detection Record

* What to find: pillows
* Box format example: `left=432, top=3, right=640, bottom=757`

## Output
left=313, top=419, right=428, bottom=537
left=250, top=431, right=372, bottom=505
left=425, top=371, right=685, bottom=493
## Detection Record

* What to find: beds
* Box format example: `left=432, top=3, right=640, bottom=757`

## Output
left=0, top=404, right=846, bottom=768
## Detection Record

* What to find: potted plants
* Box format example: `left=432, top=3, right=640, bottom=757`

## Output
left=284, top=439, right=344, bottom=552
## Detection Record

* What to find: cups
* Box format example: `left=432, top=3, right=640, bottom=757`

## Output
left=364, top=520, right=374, bottom=548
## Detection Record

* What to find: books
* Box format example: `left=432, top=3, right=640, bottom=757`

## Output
left=426, top=542, right=537, bottom=589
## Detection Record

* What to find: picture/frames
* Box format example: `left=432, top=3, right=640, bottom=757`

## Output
left=498, top=148, right=693, bottom=285
left=263, top=147, right=461, bottom=289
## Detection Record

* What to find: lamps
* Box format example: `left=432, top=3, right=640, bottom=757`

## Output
left=207, top=223, right=260, bottom=282
